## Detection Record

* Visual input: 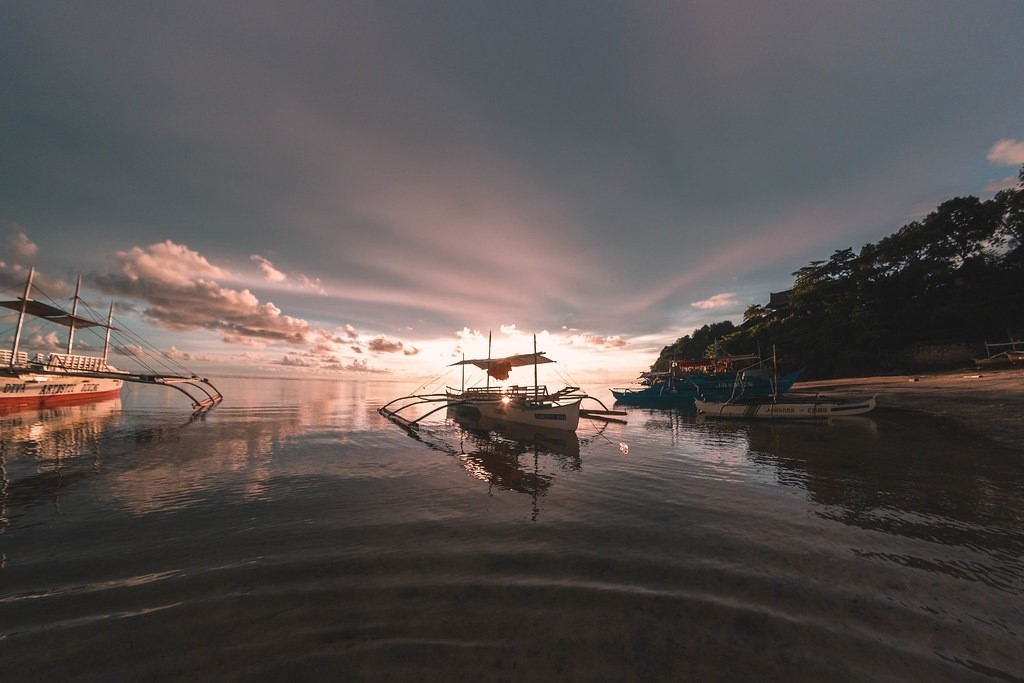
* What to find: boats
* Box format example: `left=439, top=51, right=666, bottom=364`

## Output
left=971, top=351, right=1024, bottom=371
left=607, top=335, right=880, bottom=420
left=0, top=268, right=224, bottom=417
left=379, top=328, right=628, bottom=436
